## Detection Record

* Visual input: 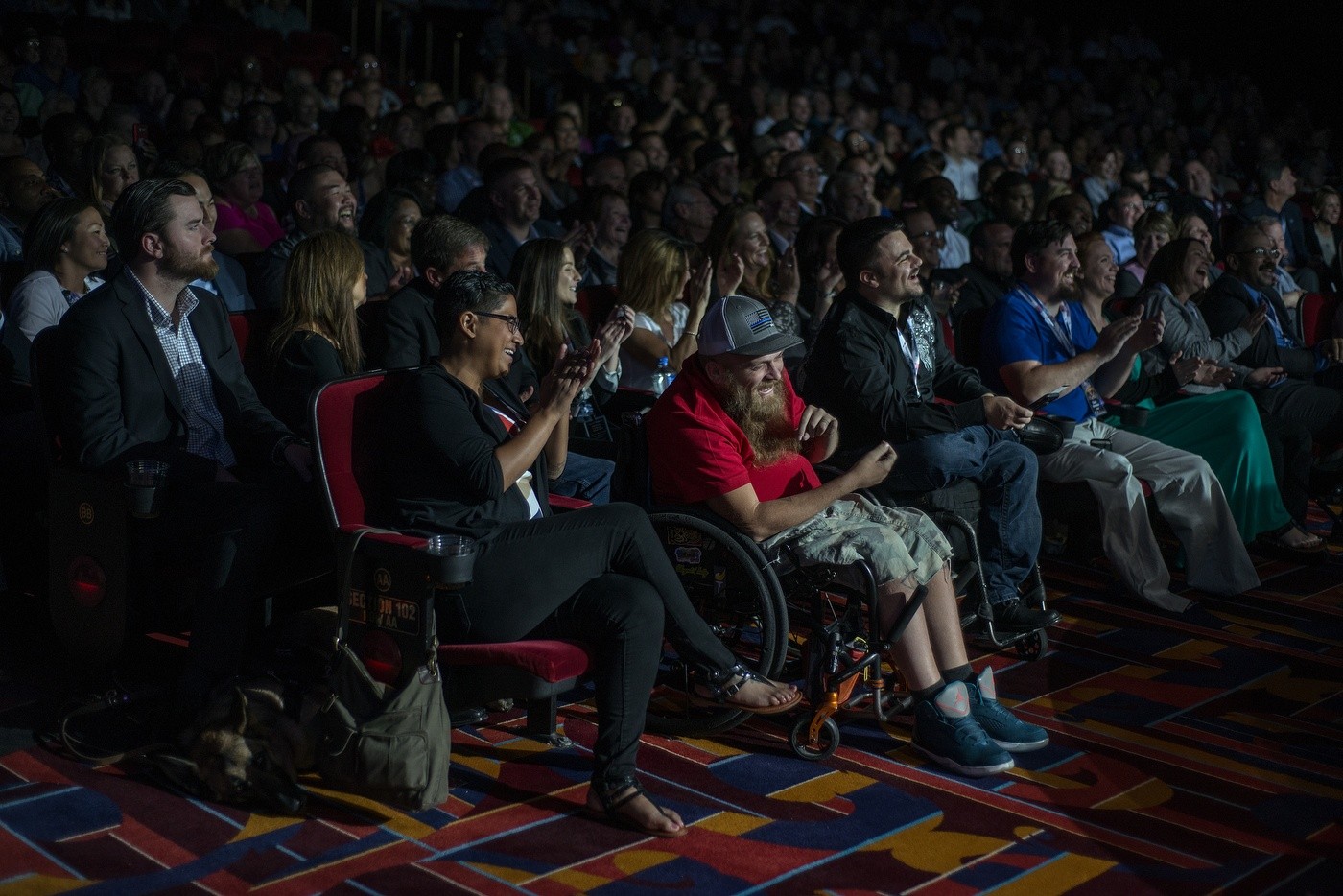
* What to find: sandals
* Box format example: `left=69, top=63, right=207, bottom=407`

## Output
left=1256, top=520, right=1329, bottom=553
left=582, top=779, right=690, bottom=837
left=688, top=662, right=802, bottom=715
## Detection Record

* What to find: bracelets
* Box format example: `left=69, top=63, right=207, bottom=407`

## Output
left=272, top=438, right=302, bottom=466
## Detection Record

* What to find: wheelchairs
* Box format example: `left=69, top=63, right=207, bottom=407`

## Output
left=569, top=409, right=984, bottom=762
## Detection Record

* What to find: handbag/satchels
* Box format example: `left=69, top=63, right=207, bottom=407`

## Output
left=295, top=641, right=456, bottom=807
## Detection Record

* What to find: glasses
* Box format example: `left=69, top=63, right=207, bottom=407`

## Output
left=240, top=62, right=262, bottom=70
left=787, top=165, right=823, bottom=177
left=387, top=215, right=418, bottom=227
left=1009, top=147, right=1029, bottom=156
left=687, top=199, right=712, bottom=210
left=473, top=310, right=522, bottom=334
left=1245, top=247, right=1281, bottom=258
left=413, top=175, right=432, bottom=184
left=358, top=62, right=382, bottom=70
left=910, top=230, right=944, bottom=240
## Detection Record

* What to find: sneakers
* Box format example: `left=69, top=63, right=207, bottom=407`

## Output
left=965, top=665, right=1050, bottom=749
left=911, top=679, right=1015, bottom=775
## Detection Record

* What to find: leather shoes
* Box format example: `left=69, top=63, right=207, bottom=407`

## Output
left=448, top=704, right=489, bottom=728
left=980, top=599, right=1062, bottom=631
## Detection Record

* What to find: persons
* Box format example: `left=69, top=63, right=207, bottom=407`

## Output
left=55, top=177, right=358, bottom=747
left=1123, top=235, right=1314, bottom=525
left=1198, top=222, right=1343, bottom=546
left=0, top=0, right=1341, bottom=503
left=804, top=216, right=1064, bottom=631
left=1054, top=228, right=1328, bottom=555
left=968, top=216, right=1260, bottom=620
left=349, top=268, right=803, bottom=838
left=652, top=293, right=1050, bottom=778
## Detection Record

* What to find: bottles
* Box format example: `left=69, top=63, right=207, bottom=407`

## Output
left=578, top=395, right=594, bottom=420
left=651, top=355, right=678, bottom=402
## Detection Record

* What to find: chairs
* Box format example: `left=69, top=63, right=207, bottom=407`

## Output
left=4, top=283, right=1152, bottom=800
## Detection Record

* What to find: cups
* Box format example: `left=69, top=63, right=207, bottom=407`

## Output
left=929, top=281, right=955, bottom=315
left=427, top=533, right=475, bottom=558
left=127, top=456, right=168, bottom=486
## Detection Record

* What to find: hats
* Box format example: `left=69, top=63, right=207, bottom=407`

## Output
left=744, top=134, right=788, bottom=164
left=696, top=295, right=805, bottom=357
left=690, top=137, right=736, bottom=173
